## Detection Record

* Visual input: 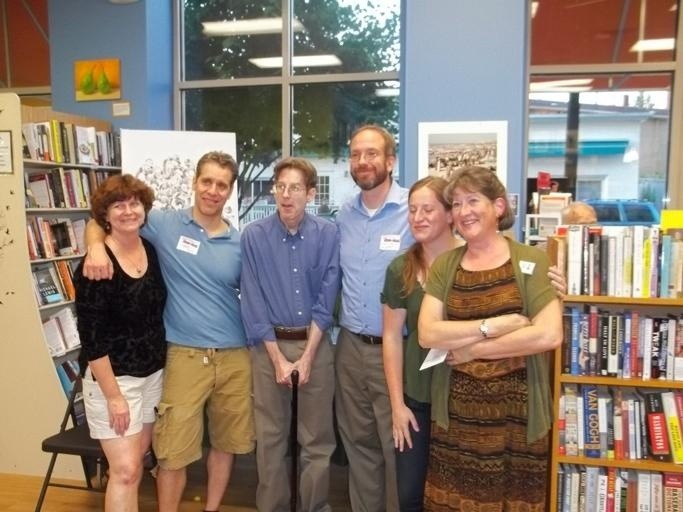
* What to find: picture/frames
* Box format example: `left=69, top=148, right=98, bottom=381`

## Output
left=417, top=120, right=507, bottom=191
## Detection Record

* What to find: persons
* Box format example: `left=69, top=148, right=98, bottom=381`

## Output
left=418, top=164, right=565, bottom=511
left=568, top=201, right=597, bottom=224
left=378, top=174, right=568, bottom=512
left=332, top=124, right=417, bottom=512
left=72, top=173, right=169, bottom=512
left=83, top=151, right=256, bottom=511
left=239, top=156, right=340, bottom=511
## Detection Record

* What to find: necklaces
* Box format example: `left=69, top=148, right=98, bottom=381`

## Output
left=110, top=238, right=144, bottom=273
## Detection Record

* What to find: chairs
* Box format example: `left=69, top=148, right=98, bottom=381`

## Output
left=32, top=376, right=157, bottom=512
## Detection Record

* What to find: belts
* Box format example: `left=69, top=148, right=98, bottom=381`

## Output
left=274, top=327, right=307, bottom=340
left=351, top=332, right=382, bottom=345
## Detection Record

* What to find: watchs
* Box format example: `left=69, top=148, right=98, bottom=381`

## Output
left=479, top=318, right=488, bottom=340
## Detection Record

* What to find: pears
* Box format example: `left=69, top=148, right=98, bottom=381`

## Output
left=80, top=66, right=111, bottom=94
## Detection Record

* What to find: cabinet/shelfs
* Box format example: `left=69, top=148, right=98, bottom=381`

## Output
left=0, top=92, right=122, bottom=481
left=545, top=235, right=682, bottom=511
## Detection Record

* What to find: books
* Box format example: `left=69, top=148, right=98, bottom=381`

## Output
left=558, top=226, right=683, bottom=512
left=528, top=177, right=573, bottom=238
left=20, top=120, right=120, bottom=396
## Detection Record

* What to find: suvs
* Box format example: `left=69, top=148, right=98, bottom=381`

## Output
left=579, top=197, right=660, bottom=229
